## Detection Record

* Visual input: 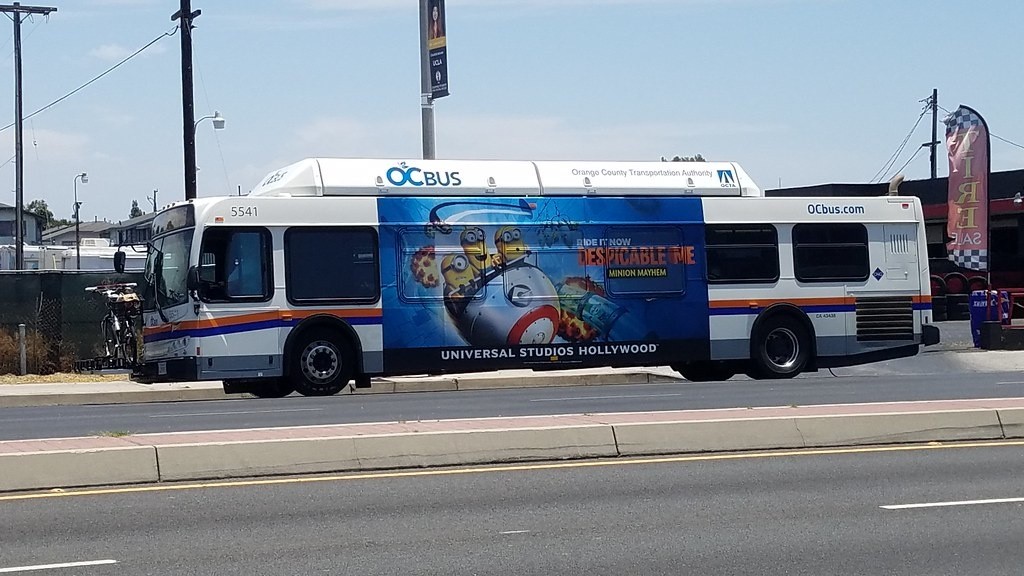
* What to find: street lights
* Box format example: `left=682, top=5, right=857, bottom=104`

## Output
left=183, top=111, right=226, bottom=201
left=147, top=188, right=158, bottom=213
left=73, top=173, right=88, bottom=268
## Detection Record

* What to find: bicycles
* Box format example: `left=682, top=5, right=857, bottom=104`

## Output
left=85, top=282, right=140, bottom=366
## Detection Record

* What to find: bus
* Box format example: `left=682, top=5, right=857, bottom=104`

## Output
left=928, top=257, right=1003, bottom=295
left=114, top=157, right=940, bottom=399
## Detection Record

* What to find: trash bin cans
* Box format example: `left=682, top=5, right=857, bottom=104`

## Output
left=980, top=320, right=1002, bottom=350
